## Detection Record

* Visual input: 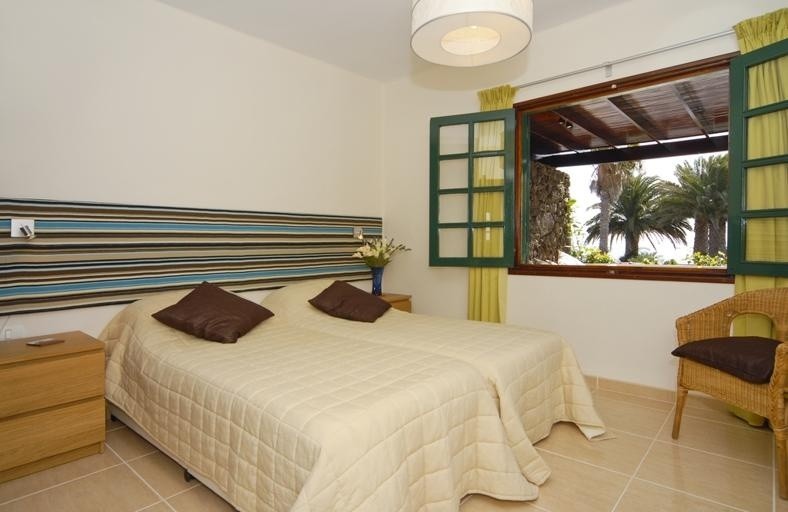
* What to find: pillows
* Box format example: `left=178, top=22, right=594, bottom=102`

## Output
left=308, top=280, right=392, bottom=322
left=151, top=281, right=274, bottom=343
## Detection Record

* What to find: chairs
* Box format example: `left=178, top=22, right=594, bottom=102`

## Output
left=670, top=288, right=788, bottom=501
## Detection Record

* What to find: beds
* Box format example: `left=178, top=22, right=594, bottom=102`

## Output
left=98, top=278, right=605, bottom=512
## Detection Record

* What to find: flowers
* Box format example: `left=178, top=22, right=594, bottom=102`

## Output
left=351, top=237, right=412, bottom=269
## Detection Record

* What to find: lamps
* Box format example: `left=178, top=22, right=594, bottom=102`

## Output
left=10, top=219, right=35, bottom=238
left=409, top=2, right=534, bottom=70
left=353, top=226, right=364, bottom=240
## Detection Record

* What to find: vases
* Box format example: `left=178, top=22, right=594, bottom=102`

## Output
left=370, top=267, right=384, bottom=295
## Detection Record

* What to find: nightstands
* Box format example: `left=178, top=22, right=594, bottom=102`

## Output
left=0, top=330, right=107, bottom=485
left=364, top=292, right=412, bottom=314
